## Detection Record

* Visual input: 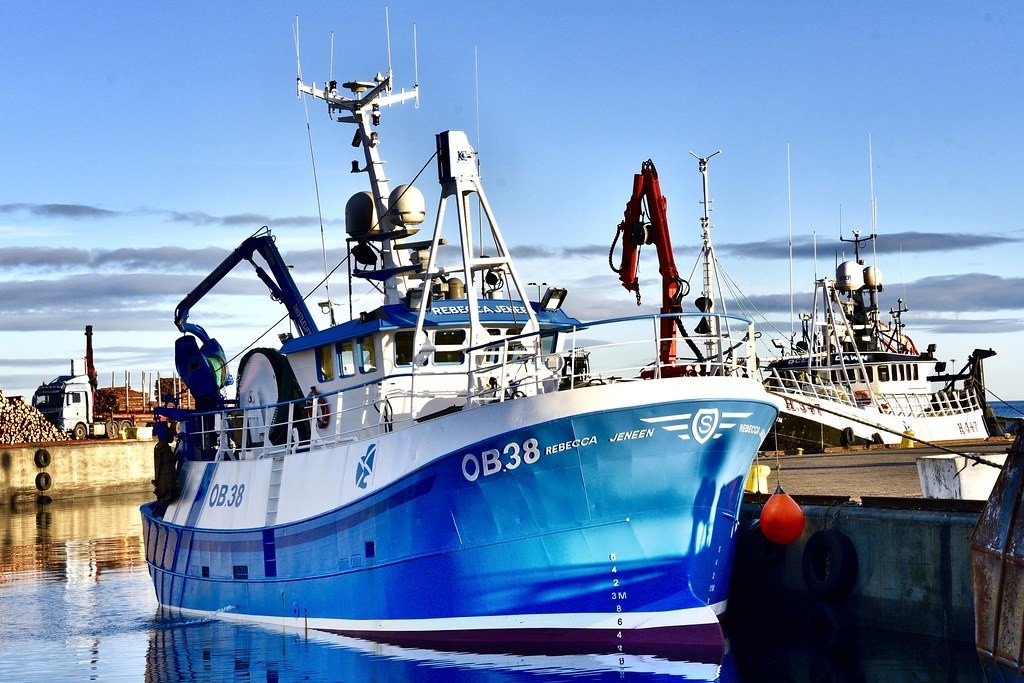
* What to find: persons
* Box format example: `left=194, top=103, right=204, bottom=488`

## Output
left=154, top=431, right=175, bottom=516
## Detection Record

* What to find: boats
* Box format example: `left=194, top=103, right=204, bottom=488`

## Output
left=640, top=130, right=1024, bottom=454
left=137, top=2, right=786, bottom=683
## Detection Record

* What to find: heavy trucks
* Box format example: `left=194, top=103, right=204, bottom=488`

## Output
left=30, top=374, right=153, bottom=440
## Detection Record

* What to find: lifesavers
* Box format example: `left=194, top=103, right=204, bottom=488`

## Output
left=873, top=432, right=884, bottom=444
left=305, top=390, right=330, bottom=429
left=36, top=512, right=53, bottom=529
left=34, top=449, right=51, bottom=468
left=37, top=495, right=52, bottom=504
left=35, top=472, right=51, bottom=491
left=801, top=528, right=859, bottom=602
left=854, top=391, right=870, bottom=406
left=841, top=426, right=854, bottom=445
